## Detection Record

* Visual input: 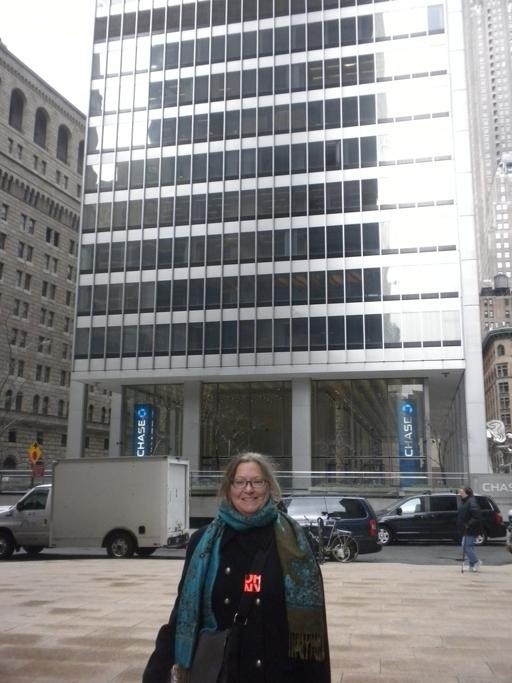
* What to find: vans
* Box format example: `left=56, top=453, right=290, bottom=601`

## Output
left=374, top=489, right=506, bottom=546
left=278, top=496, right=383, bottom=562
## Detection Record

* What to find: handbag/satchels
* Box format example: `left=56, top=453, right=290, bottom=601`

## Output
left=187, top=625, right=245, bottom=682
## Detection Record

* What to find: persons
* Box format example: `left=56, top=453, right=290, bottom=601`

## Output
left=454, top=486, right=482, bottom=572
left=139, top=450, right=336, bottom=683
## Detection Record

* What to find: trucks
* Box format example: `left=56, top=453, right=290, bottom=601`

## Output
left=2, top=454, right=191, bottom=560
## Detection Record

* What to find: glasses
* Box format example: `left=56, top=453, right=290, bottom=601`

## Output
left=231, top=479, right=271, bottom=488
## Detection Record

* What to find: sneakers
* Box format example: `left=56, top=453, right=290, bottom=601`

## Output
left=472, top=559, right=482, bottom=572
